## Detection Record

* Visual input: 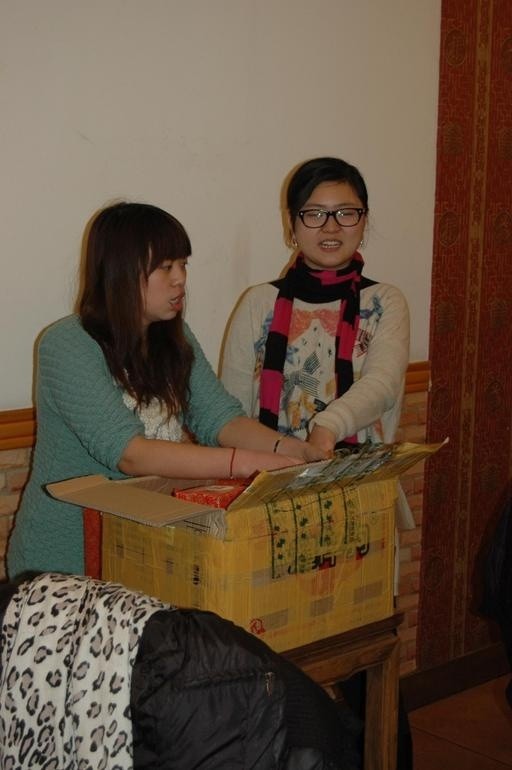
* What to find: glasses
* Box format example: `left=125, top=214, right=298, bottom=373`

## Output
left=296, top=208, right=366, bottom=228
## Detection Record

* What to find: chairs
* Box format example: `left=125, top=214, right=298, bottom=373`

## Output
left=0, top=570, right=361, bottom=770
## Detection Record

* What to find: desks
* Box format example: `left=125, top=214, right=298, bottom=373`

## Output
left=276, top=608, right=405, bottom=770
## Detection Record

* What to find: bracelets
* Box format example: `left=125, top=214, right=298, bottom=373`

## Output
left=230, top=445, right=236, bottom=479
left=272, top=433, right=289, bottom=453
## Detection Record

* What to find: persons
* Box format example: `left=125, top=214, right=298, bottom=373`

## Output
left=4, top=203, right=331, bottom=588
left=217, top=156, right=415, bottom=769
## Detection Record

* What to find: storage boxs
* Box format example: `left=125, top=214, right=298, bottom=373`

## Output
left=46, top=435, right=450, bottom=655
